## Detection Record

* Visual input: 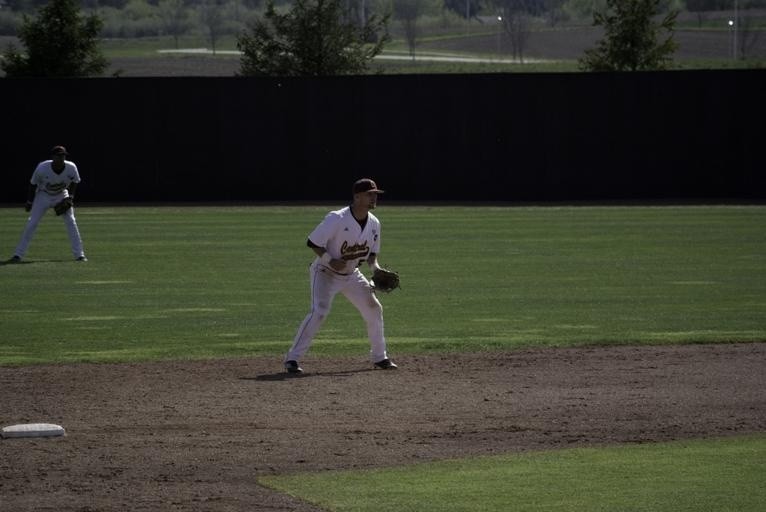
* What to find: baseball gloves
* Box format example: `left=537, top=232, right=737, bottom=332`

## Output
left=54, top=196, right=72, bottom=214
left=371, top=268, right=399, bottom=293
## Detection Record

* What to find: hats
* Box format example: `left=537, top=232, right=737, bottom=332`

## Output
left=353, top=179, right=384, bottom=194
left=53, top=146, right=68, bottom=155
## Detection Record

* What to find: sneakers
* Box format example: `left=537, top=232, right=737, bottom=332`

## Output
left=11, top=255, right=21, bottom=264
left=374, top=358, right=398, bottom=370
left=76, top=256, right=88, bottom=261
left=283, top=360, right=304, bottom=375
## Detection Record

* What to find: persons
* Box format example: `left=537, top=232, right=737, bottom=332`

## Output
left=283, top=178, right=399, bottom=373
left=13, top=145, right=88, bottom=262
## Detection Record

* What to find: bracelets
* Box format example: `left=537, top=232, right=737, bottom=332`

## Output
left=370, top=263, right=380, bottom=273
left=27, top=200, right=33, bottom=205
left=321, top=253, right=332, bottom=263
